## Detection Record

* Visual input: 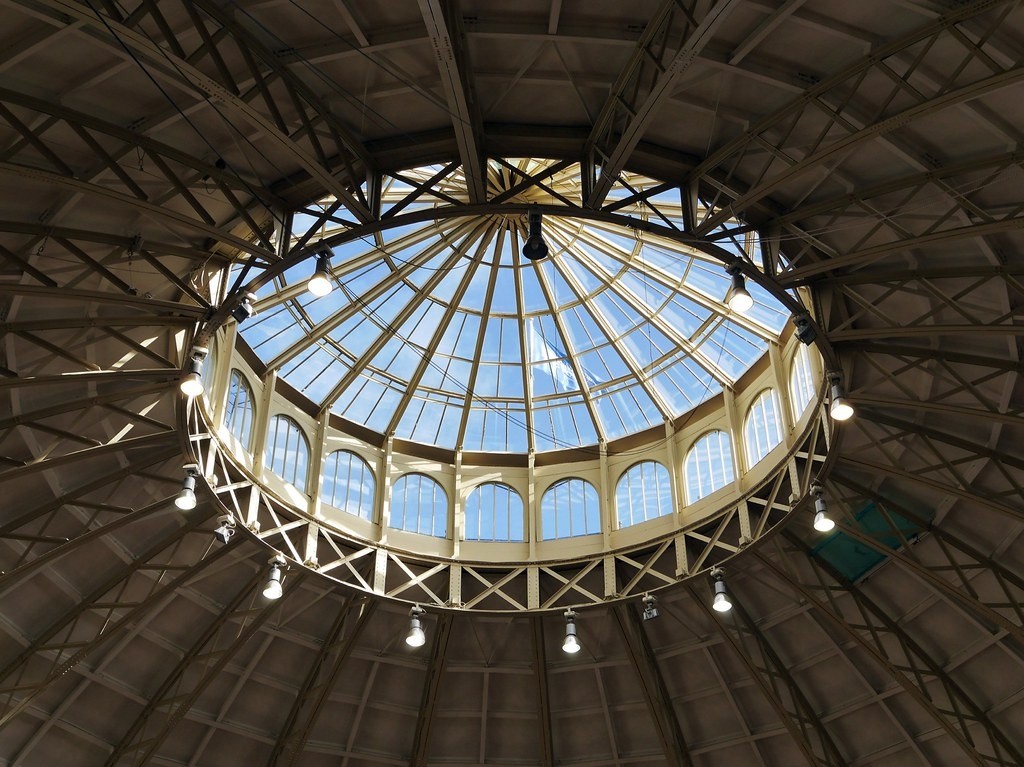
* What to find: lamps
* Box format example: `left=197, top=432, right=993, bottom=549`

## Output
left=406, top=606, right=428, bottom=647
left=710, top=568, right=733, bottom=612
left=642, top=595, right=660, bottom=620
left=792, top=311, right=816, bottom=345
left=308, top=244, right=335, bottom=295
left=724, top=257, right=754, bottom=313
left=180, top=345, right=209, bottom=396
left=263, top=555, right=287, bottom=599
left=214, top=514, right=236, bottom=545
left=522, top=201, right=548, bottom=260
left=231, top=293, right=257, bottom=324
left=809, top=486, right=835, bottom=532
left=562, top=611, right=580, bottom=654
left=175, top=463, right=200, bottom=510
left=825, top=369, right=854, bottom=421
